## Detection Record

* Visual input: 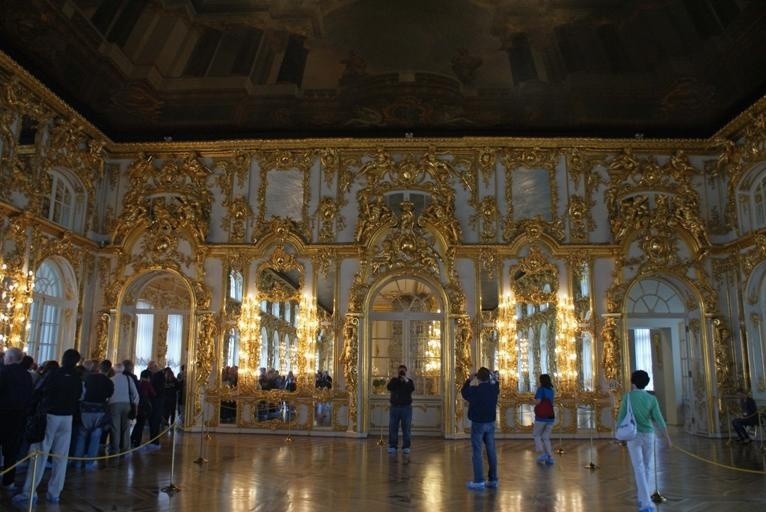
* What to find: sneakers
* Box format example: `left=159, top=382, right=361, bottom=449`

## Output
left=466, top=481, right=485, bottom=490
left=387, top=448, right=396, bottom=454
left=4, top=484, right=60, bottom=504
left=485, top=480, right=497, bottom=488
left=402, top=448, right=410, bottom=454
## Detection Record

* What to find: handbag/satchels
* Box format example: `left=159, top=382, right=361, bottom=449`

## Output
left=128, top=403, right=136, bottom=420
left=27, top=413, right=47, bottom=444
left=615, top=413, right=638, bottom=441
left=534, top=400, right=554, bottom=417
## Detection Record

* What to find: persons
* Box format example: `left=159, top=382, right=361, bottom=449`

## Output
left=2, top=348, right=186, bottom=508
left=220, top=366, right=238, bottom=424
left=387, top=364, right=415, bottom=455
left=733, top=388, right=759, bottom=445
left=314, top=370, right=330, bottom=427
left=614, top=370, right=673, bottom=512
left=254, top=367, right=297, bottom=423
left=461, top=366, right=499, bottom=491
left=529, top=374, right=555, bottom=465
